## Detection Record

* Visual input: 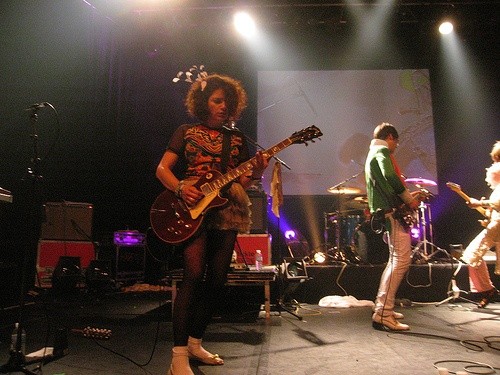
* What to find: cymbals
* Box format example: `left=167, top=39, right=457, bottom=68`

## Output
left=404, top=177, right=437, bottom=186
left=326, top=187, right=360, bottom=195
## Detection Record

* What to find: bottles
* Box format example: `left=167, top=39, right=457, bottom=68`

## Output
left=11, top=322, right=25, bottom=355
left=255, top=250, right=263, bottom=270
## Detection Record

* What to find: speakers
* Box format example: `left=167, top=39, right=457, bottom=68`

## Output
left=40, top=202, right=94, bottom=241
left=50, top=257, right=83, bottom=291
left=247, top=191, right=269, bottom=235
left=86, top=259, right=113, bottom=293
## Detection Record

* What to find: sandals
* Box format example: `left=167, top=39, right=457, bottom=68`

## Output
left=188, top=339, right=223, bottom=365
left=168, top=349, right=188, bottom=375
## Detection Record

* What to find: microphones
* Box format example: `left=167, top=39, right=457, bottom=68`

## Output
left=23, top=102, right=47, bottom=112
left=229, top=120, right=236, bottom=130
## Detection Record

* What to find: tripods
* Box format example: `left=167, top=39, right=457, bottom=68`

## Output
left=261, top=208, right=304, bottom=321
left=409, top=186, right=455, bottom=265
left=306, top=214, right=349, bottom=266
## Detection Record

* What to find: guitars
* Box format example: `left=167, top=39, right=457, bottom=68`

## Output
left=446, top=181, right=489, bottom=218
left=150, top=125, right=323, bottom=244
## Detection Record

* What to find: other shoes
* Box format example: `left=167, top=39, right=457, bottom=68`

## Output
left=477, top=288, right=498, bottom=307
left=392, top=311, right=404, bottom=319
left=372, top=312, right=410, bottom=331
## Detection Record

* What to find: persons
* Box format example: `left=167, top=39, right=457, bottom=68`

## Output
left=155, top=75, right=272, bottom=375
left=466, top=142, right=500, bottom=307
left=365, top=123, right=426, bottom=330
